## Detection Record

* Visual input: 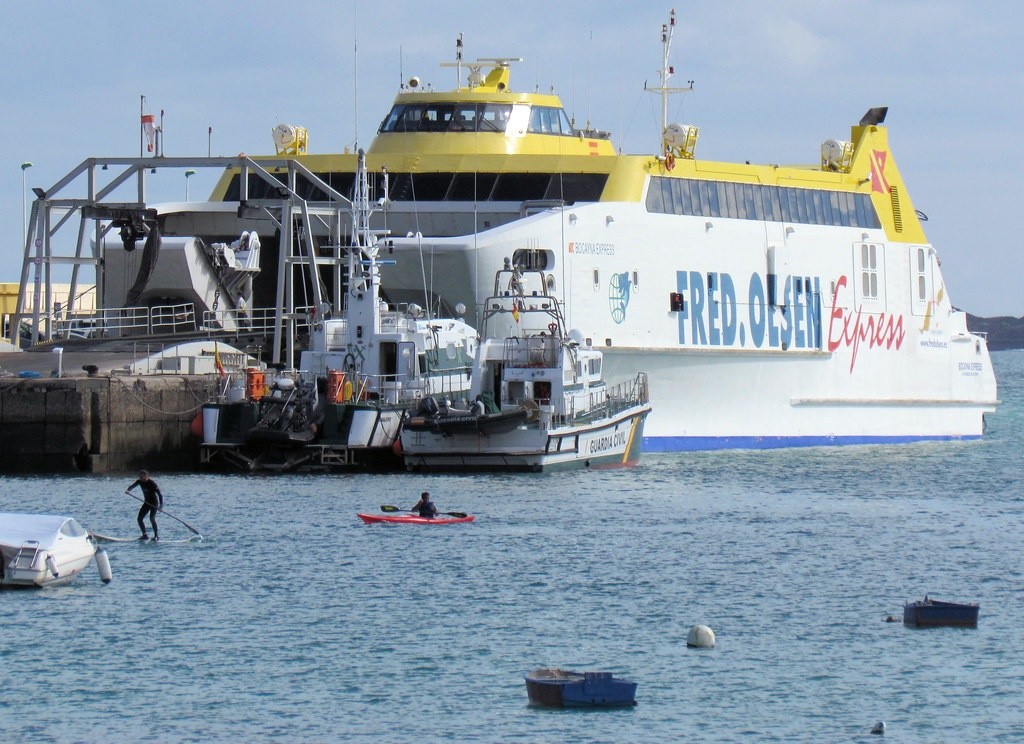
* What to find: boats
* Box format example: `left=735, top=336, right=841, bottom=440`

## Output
left=522, top=669, right=637, bottom=710
left=354, top=509, right=476, bottom=528
left=0, top=511, right=113, bottom=589
left=391, top=238, right=654, bottom=478
left=88, top=5, right=1001, bottom=443
left=903, top=596, right=981, bottom=630
left=189, top=145, right=488, bottom=473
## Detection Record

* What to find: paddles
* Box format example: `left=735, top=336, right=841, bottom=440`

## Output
left=375, top=502, right=469, bottom=519
left=121, top=492, right=197, bottom=534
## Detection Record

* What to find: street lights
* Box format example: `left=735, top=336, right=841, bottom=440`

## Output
left=21, top=162, right=33, bottom=249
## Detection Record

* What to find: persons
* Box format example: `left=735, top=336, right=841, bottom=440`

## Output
left=470, top=394, right=487, bottom=415
left=411, top=492, right=440, bottom=519
left=125, top=470, right=165, bottom=543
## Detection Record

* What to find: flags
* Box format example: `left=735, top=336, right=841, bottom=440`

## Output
left=511, top=304, right=520, bottom=324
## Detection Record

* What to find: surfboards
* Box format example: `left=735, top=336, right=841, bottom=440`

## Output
left=89, top=529, right=203, bottom=547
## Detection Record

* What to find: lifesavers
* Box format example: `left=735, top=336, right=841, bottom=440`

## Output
left=664, top=152, right=677, bottom=171
